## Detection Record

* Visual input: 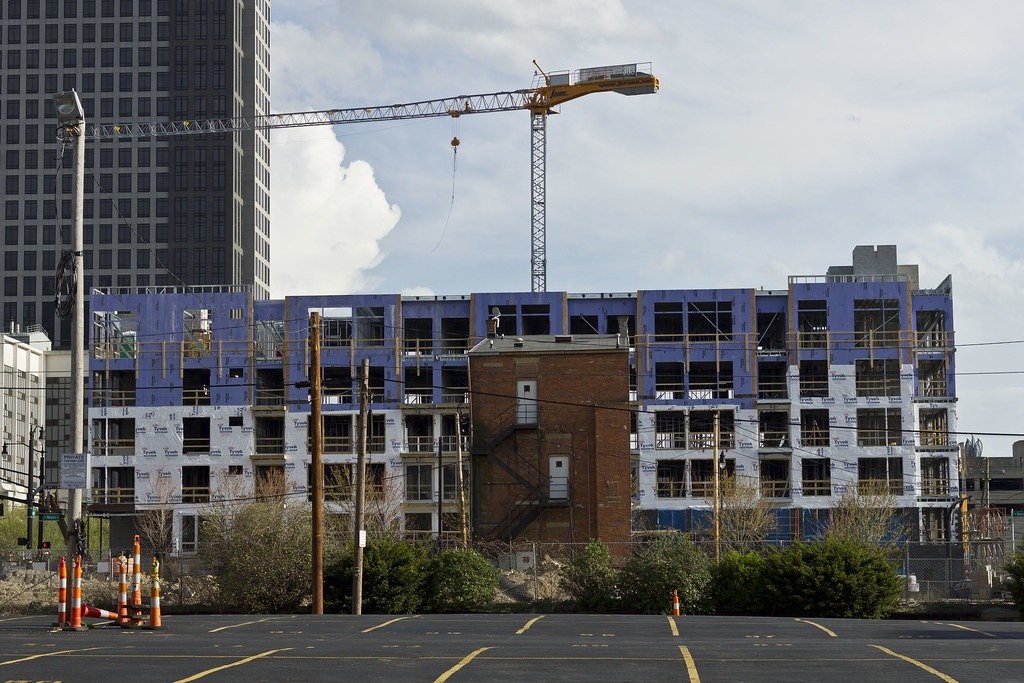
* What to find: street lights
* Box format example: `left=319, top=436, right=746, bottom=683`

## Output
left=54, top=90, right=87, bottom=624
left=720, top=451, right=727, bottom=510
left=2, top=440, right=46, bottom=549
left=27, top=424, right=46, bottom=549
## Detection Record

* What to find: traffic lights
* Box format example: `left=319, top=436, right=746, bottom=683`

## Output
left=42, top=541, right=49, bottom=550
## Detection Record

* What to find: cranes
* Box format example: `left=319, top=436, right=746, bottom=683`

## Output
left=59, top=59, right=659, bottom=293
